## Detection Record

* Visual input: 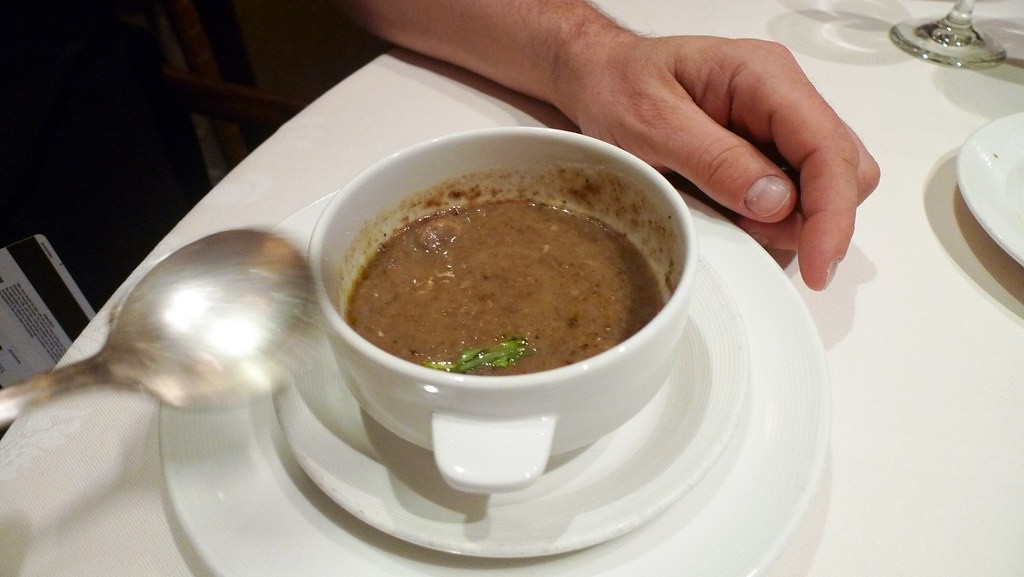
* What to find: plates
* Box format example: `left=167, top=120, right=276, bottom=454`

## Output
left=957, top=111, right=1024, bottom=271
left=157, top=188, right=830, bottom=577
left=274, top=189, right=752, bottom=559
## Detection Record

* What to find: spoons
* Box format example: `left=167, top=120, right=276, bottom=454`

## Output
left=0, top=228, right=317, bottom=427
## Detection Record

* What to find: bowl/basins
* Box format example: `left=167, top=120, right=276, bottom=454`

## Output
left=307, top=127, right=696, bottom=492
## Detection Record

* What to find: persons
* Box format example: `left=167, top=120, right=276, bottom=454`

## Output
left=0, top=0, right=881, bottom=322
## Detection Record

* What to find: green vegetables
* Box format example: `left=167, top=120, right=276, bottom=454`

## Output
left=424, top=336, right=530, bottom=374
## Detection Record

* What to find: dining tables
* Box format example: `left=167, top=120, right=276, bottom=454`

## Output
left=0, top=0, right=1024, bottom=577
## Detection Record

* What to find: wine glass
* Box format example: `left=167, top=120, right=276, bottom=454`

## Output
left=890, top=0, right=1007, bottom=68
